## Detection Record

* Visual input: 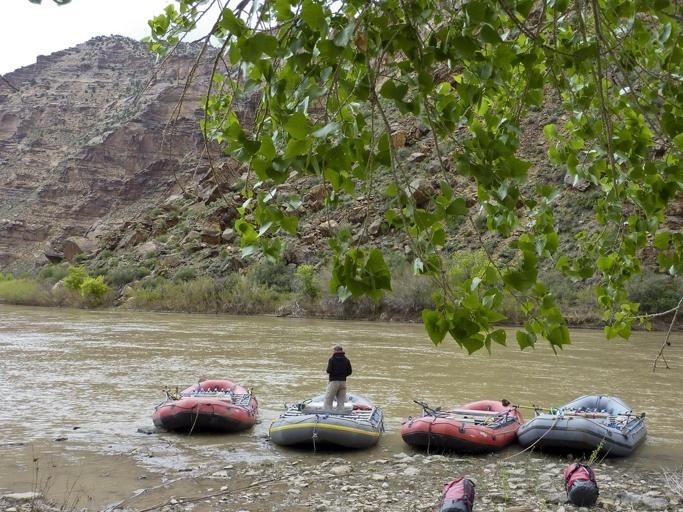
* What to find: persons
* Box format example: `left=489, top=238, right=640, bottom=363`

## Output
left=325, top=344, right=352, bottom=410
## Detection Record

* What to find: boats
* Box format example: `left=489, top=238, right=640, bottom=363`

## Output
left=150, top=378, right=262, bottom=434
left=270, top=388, right=385, bottom=451
left=399, top=398, right=525, bottom=456
left=514, top=394, right=648, bottom=462
